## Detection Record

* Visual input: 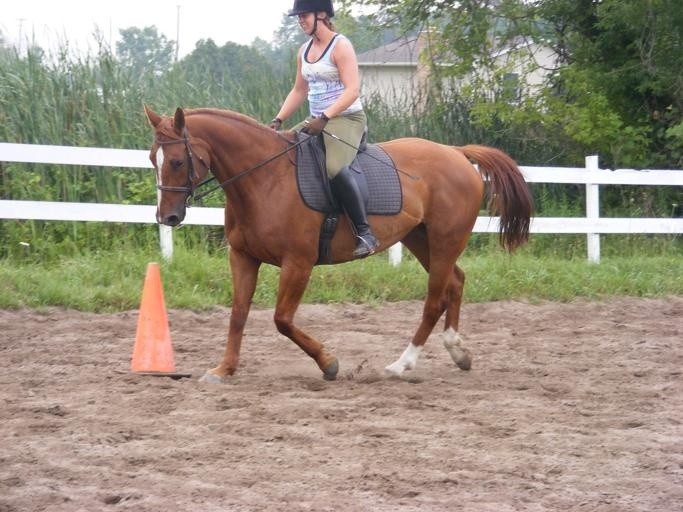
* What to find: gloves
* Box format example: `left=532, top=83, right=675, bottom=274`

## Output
left=305, top=114, right=328, bottom=134
left=268, top=118, right=280, bottom=130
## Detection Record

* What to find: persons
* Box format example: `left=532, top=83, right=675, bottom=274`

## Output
left=267, top=0, right=382, bottom=260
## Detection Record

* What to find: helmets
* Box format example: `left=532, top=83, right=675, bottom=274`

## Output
left=289, top=0, right=334, bottom=16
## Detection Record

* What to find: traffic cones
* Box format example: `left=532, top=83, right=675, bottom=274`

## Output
left=112, top=262, right=192, bottom=378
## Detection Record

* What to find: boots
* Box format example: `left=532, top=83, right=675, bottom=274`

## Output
left=332, top=165, right=380, bottom=255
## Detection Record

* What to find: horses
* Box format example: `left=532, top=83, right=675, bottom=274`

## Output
left=143, top=102, right=536, bottom=386
